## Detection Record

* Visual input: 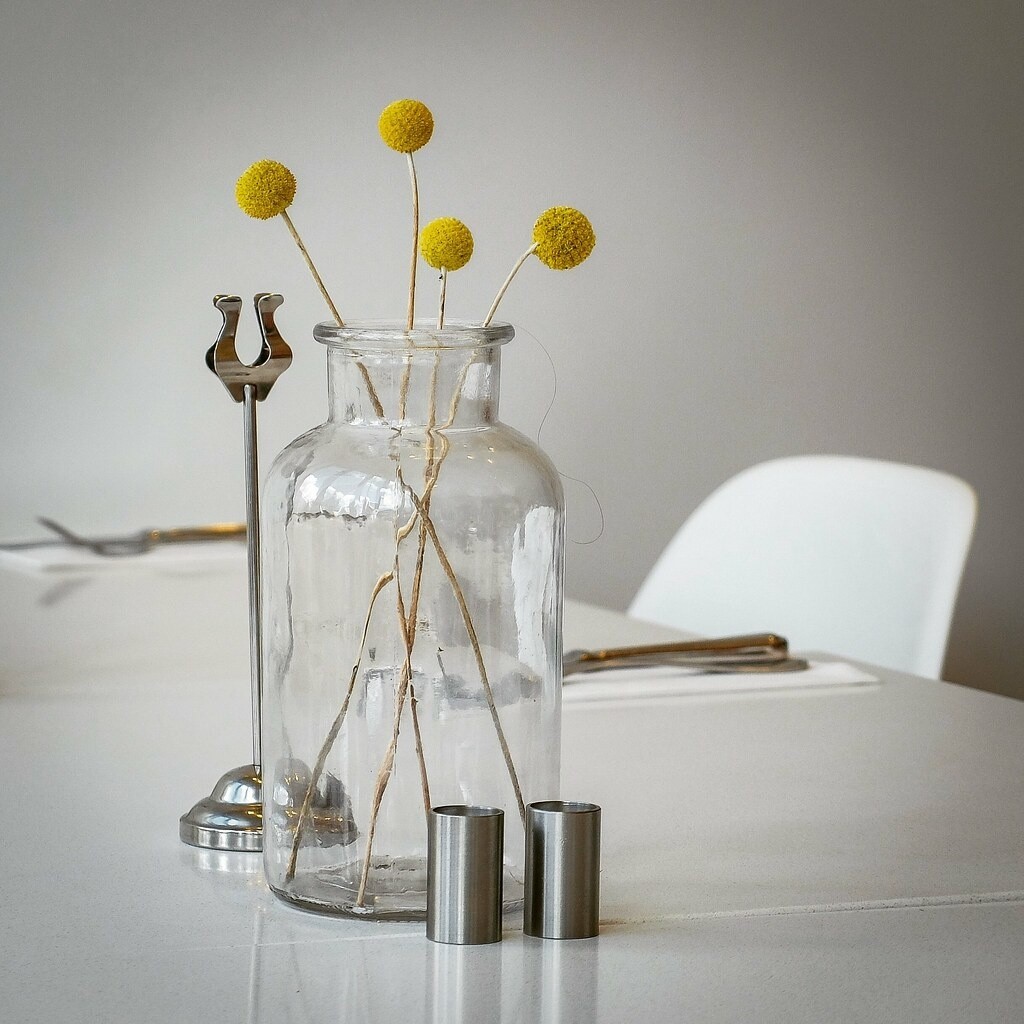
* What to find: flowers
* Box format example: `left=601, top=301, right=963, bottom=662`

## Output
left=231, top=98, right=596, bottom=906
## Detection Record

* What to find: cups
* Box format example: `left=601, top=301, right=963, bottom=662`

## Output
left=426, top=804, right=504, bottom=945
left=523, top=801, right=601, bottom=940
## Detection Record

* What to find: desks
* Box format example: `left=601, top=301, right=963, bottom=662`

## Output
left=1, top=527, right=1024, bottom=1024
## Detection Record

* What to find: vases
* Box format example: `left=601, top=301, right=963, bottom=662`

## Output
left=255, top=318, right=567, bottom=923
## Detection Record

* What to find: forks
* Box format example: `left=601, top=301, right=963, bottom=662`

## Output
left=37, top=514, right=247, bottom=557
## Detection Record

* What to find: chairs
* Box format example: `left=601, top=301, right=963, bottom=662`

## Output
left=623, top=451, right=983, bottom=682
